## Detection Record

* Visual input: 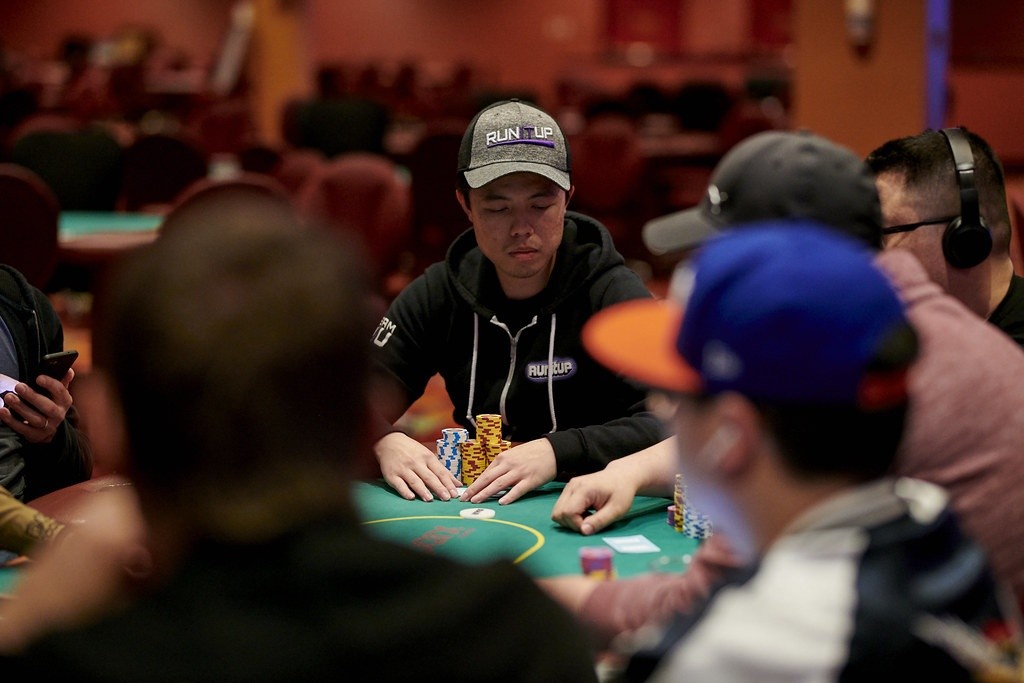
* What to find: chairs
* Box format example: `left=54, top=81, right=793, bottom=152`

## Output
left=567, top=118, right=641, bottom=247
left=0, top=162, right=62, bottom=294
left=298, top=155, right=411, bottom=271
left=171, top=174, right=285, bottom=212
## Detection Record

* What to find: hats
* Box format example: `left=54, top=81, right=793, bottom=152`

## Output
left=584, top=223, right=917, bottom=412
left=644, top=132, right=881, bottom=257
left=456, top=98, right=574, bottom=192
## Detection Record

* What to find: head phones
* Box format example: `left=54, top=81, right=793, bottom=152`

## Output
left=936, top=127, right=993, bottom=271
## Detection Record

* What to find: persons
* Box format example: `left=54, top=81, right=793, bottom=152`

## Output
left=368, top=97, right=678, bottom=505
left=0, top=487, right=70, bottom=561
left=581, top=228, right=1023, bottom=683
left=0, top=264, right=93, bottom=501
left=0, top=210, right=599, bottom=683
left=551, top=132, right=1024, bottom=591
left=863, top=125, right=1024, bottom=349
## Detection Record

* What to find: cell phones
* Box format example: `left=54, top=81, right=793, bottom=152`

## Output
left=9, top=349, right=80, bottom=425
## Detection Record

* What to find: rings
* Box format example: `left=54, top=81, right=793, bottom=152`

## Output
left=43, top=420, right=48, bottom=430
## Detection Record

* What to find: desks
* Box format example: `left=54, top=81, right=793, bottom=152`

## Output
left=59, top=209, right=167, bottom=267
left=0, top=480, right=701, bottom=597
